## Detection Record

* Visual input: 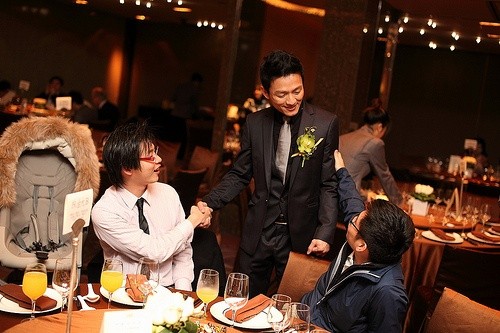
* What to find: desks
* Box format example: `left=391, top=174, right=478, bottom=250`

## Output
left=0, top=284, right=328, bottom=333
left=334, top=195, right=500, bottom=333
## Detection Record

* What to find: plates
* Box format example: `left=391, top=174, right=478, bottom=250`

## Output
left=210, top=298, right=284, bottom=329
left=99, top=279, right=172, bottom=306
left=466, top=222, right=500, bottom=244
left=421, top=231, right=464, bottom=243
left=0, top=285, right=67, bottom=314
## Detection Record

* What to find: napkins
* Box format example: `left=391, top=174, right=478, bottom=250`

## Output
left=225, top=295, right=272, bottom=324
left=0, top=284, right=56, bottom=311
left=471, top=231, right=500, bottom=242
left=125, top=273, right=154, bottom=303
left=431, top=228, right=455, bottom=241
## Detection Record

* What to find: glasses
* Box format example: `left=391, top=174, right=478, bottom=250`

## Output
left=349, top=213, right=365, bottom=238
left=139, top=144, right=160, bottom=162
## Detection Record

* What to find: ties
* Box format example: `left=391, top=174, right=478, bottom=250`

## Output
left=136, top=198, right=150, bottom=280
left=275, top=116, right=291, bottom=185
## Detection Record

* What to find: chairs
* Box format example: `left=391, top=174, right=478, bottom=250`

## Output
left=424, top=245, right=500, bottom=333
left=276, top=252, right=331, bottom=310
left=4, top=103, right=228, bottom=293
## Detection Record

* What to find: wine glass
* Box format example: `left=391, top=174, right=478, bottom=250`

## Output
left=224, top=273, right=249, bottom=329
left=267, top=294, right=292, bottom=333
left=196, top=269, right=219, bottom=320
left=434, top=188, right=454, bottom=213
left=20, top=263, right=47, bottom=324
left=52, top=258, right=78, bottom=313
left=100, top=258, right=124, bottom=309
left=136, top=256, right=160, bottom=309
left=456, top=202, right=491, bottom=239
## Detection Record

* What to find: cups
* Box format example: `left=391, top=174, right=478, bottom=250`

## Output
left=282, top=302, right=310, bottom=333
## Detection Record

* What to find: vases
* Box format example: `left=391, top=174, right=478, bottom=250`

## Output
left=411, top=200, right=432, bottom=216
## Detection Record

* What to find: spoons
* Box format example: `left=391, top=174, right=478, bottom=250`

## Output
left=76, top=283, right=100, bottom=311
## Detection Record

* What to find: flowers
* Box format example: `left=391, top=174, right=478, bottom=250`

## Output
left=413, top=184, right=437, bottom=206
left=145, top=290, right=204, bottom=333
left=291, top=127, right=325, bottom=167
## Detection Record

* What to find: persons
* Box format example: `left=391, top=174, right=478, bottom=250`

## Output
left=297, top=150, right=415, bottom=333
left=243, top=85, right=271, bottom=113
left=83, top=87, right=118, bottom=129
left=339, top=98, right=412, bottom=215
left=38, top=77, right=65, bottom=105
left=468, top=137, right=493, bottom=172
left=169, top=72, right=205, bottom=150
left=197, top=50, right=340, bottom=302
left=92, top=118, right=213, bottom=291
